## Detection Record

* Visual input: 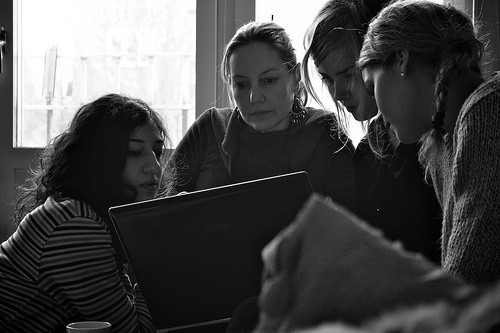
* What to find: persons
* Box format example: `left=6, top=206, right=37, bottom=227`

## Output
left=1, top=92, right=165, bottom=332
left=156, top=21, right=355, bottom=208
left=360, top=0, right=500, bottom=288
left=301, top=0, right=439, bottom=265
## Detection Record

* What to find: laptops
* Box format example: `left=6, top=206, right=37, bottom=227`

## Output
left=108, top=170, right=314, bottom=333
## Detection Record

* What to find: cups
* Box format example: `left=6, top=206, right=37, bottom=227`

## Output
left=66, top=321, right=112, bottom=333
